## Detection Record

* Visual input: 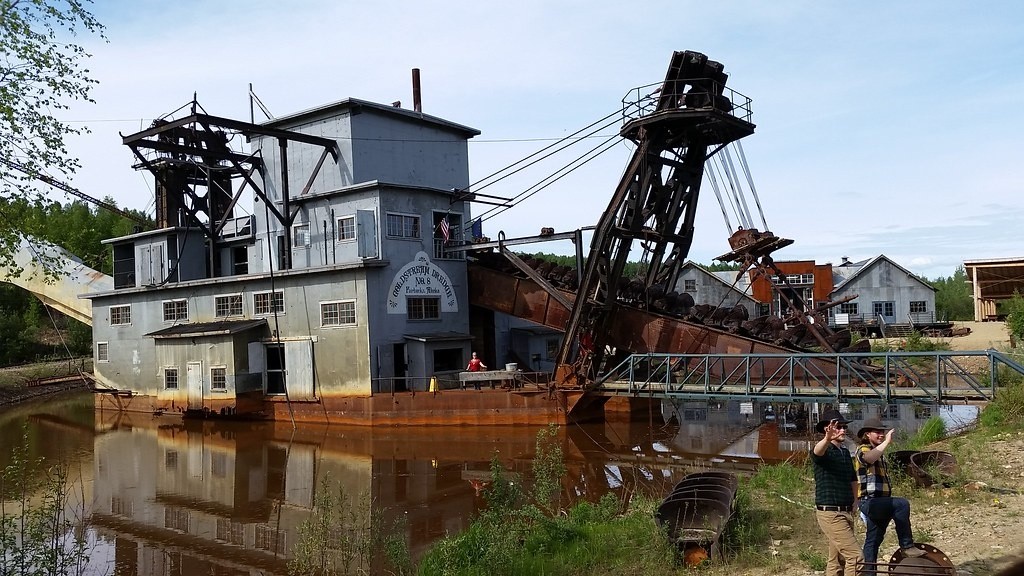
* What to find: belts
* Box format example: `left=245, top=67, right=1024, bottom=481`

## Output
left=817, top=506, right=854, bottom=511
left=862, top=495, right=870, bottom=500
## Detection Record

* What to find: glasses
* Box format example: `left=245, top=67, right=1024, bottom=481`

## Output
left=835, top=424, right=848, bottom=430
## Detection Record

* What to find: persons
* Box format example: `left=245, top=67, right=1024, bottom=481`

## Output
left=466, top=352, right=487, bottom=390
left=809, top=410, right=867, bottom=576
left=855, top=417, right=929, bottom=576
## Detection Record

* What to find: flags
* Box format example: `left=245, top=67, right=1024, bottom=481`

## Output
left=472, top=218, right=482, bottom=239
left=440, top=213, right=451, bottom=243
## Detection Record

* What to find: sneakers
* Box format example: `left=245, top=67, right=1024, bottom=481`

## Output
left=901, top=546, right=926, bottom=557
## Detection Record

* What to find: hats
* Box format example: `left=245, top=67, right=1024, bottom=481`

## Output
left=816, top=410, right=853, bottom=434
left=857, top=418, right=892, bottom=438
